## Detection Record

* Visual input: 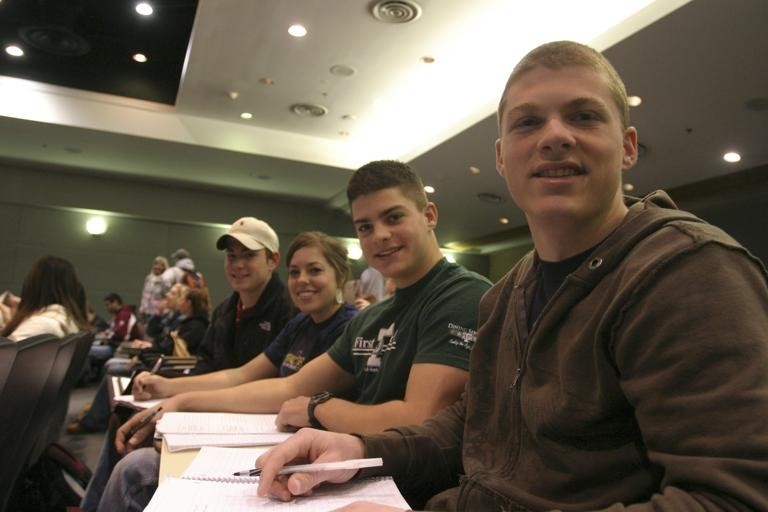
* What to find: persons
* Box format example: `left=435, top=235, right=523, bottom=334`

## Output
left=254, top=40, right=768, bottom=512
left=0, top=245, right=211, bottom=434
left=130, top=229, right=363, bottom=401
left=115, top=159, right=496, bottom=512
left=157, top=216, right=295, bottom=379
left=343, top=265, right=397, bottom=311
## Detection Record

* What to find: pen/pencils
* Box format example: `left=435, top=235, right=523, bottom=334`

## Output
left=124, top=407, right=162, bottom=444
left=231, top=457, right=383, bottom=476
left=142, top=353, right=166, bottom=391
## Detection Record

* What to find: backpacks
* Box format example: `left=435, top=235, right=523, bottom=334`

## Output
left=36, top=444, right=93, bottom=508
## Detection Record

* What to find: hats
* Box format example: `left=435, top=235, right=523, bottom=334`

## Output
left=217, top=217, right=280, bottom=255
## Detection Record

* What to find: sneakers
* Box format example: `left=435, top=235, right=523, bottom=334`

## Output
left=66, top=421, right=93, bottom=432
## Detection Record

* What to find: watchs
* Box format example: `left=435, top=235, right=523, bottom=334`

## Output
left=307, top=389, right=336, bottom=431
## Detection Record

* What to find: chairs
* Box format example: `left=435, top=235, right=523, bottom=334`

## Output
left=0, top=328, right=96, bottom=512
